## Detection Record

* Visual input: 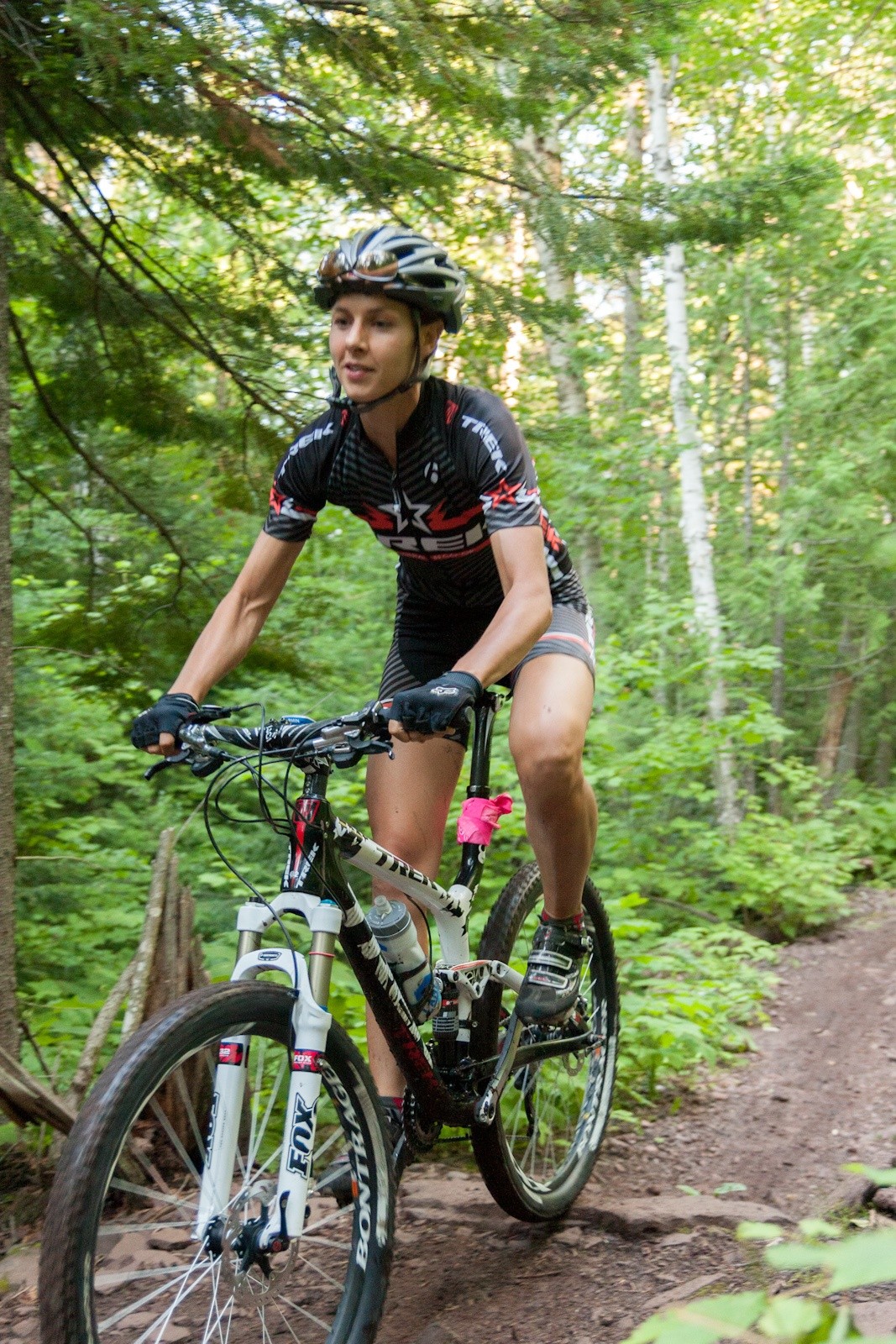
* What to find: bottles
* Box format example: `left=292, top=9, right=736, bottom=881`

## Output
left=366, top=894, right=441, bottom=1024
left=430, top=961, right=463, bottom=1070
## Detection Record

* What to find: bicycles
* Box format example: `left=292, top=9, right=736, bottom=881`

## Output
left=35, top=689, right=626, bottom=1344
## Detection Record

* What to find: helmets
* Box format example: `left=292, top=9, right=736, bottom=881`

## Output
left=315, top=225, right=466, bottom=334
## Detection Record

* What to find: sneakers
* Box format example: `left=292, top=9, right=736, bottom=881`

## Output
left=515, top=924, right=593, bottom=1020
left=318, top=1098, right=403, bottom=1194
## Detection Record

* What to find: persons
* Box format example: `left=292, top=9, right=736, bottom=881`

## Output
left=125, top=225, right=594, bottom=1193
